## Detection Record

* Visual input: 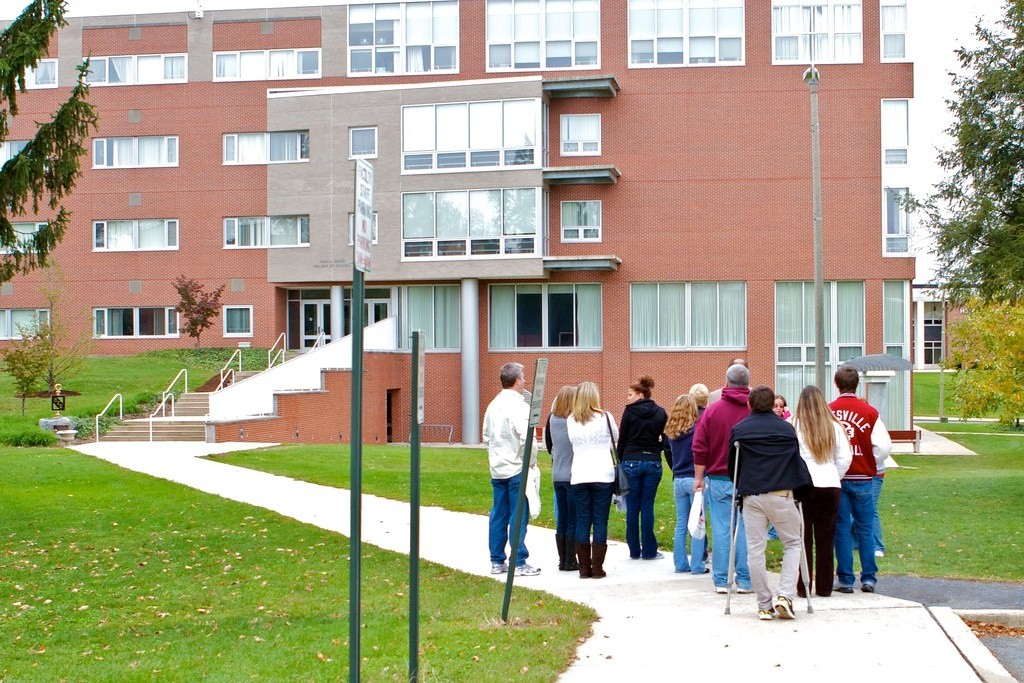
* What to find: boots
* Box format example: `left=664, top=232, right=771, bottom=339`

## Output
left=577, top=543, right=592, bottom=578
left=591, top=543, right=608, bottom=579
left=555, top=534, right=579, bottom=571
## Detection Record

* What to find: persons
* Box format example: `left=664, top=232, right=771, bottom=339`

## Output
left=727, top=384, right=815, bottom=621
left=708, top=358, right=755, bottom=405
left=483, top=362, right=541, bottom=577
left=663, top=394, right=710, bottom=576
left=784, top=385, right=854, bottom=597
left=692, top=364, right=755, bottom=593
left=772, top=394, right=791, bottom=420
left=566, top=381, right=620, bottom=579
left=544, top=385, right=579, bottom=571
left=689, top=383, right=710, bottom=565
left=849, top=398, right=885, bottom=557
left=827, top=368, right=894, bottom=594
left=618, top=375, right=672, bottom=561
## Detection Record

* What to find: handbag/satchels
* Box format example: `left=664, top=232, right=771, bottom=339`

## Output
left=687, top=483, right=710, bottom=540
left=613, top=464, right=631, bottom=497
left=524, top=463, right=543, bottom=520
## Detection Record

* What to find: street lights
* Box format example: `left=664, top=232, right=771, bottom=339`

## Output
left=802, top=68, right=828, bottom=404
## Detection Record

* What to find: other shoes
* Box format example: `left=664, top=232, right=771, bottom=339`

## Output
left=713, top=586, right=727, bottom=594
left=704, top=568, right=710, bottom=574
left=676, top=566, right=691, bottom=573
left=640, top=550, right=664, bottom=560
left=874, top=550, right=885, bottom=558
left=833, top=582, right=853, bottom=593
left=862, top=584, right=874, bottom=593
left=736, top=587, right=755, bottom=594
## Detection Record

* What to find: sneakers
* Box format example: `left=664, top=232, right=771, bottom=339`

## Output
left=513, top=564, right=541, bottom=577
left=491, top=562, right=509, bottom=574
left=774, top=596, right=796, bottom=619
left=759, top=607, right=776, bottom=619
